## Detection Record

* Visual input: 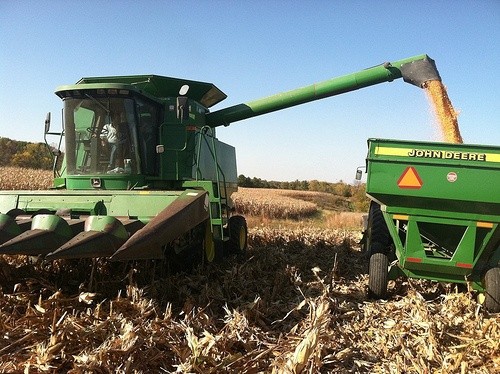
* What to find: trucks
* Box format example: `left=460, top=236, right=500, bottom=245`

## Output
left=356, top=137, right=500, bottom=313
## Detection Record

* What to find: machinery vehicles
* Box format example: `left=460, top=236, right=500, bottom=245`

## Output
left=1, top=54, right=441, bottom=266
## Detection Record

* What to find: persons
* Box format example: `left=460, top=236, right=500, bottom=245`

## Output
left=92, top=113, right=127, bottom=171
